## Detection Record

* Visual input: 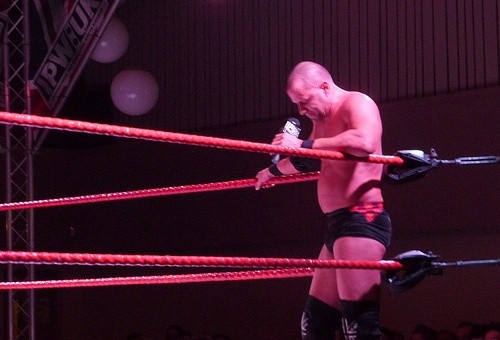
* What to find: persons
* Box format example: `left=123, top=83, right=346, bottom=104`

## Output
left=256, top=61, right=392, bottom=340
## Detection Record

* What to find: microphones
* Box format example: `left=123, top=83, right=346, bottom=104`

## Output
left=270, top=117, right=300, bottom=164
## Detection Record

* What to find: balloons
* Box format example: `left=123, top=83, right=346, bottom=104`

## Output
left=90, top=16, right=130, bottom=64
left=110, top=70, right=160, bottom=116
